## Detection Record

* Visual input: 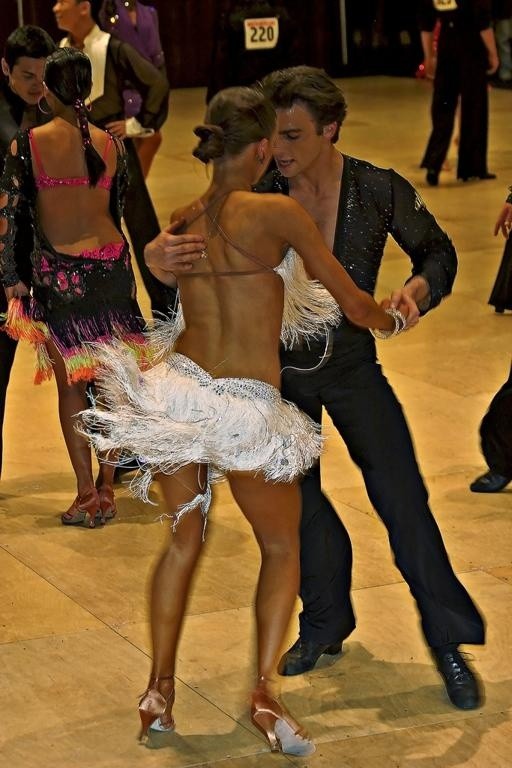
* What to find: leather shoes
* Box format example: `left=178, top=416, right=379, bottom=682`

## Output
left=430, top=635, right=481, bottom=711
left=469, top=456, right=512, bottom=493
left=277, top=619, right=348, bottom=676
left=421, top=139, right=497, bottom=185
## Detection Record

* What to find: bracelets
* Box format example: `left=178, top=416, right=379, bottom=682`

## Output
left=368, top=311, right=400, bottom=341
left=386, top=308, right=407, bottom=335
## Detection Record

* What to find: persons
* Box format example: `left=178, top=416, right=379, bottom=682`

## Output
left=207, top=0, right=313, bottom=174
left=52, top=0, right=180, bottom=333
left=0, top=47, right=153, bottom=528
left=144, top=65, right=485, bottom=710
left=0, top=26, right=146, bottom=484
left=100, top=0, right=168, bottom=183
left=420, top=1, right=511, bottom=188
left=71, top=87, right=409, bottom=757
left=470, top=187, right=512, bottom=492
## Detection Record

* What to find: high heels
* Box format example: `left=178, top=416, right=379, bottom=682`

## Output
left=95, top=485, right=119, bottom=524
left=249, top=680, right=318, bottom=757
left=135, top=671, right=176, bottom=741
left=61, top=489, right=100, bottom=527
